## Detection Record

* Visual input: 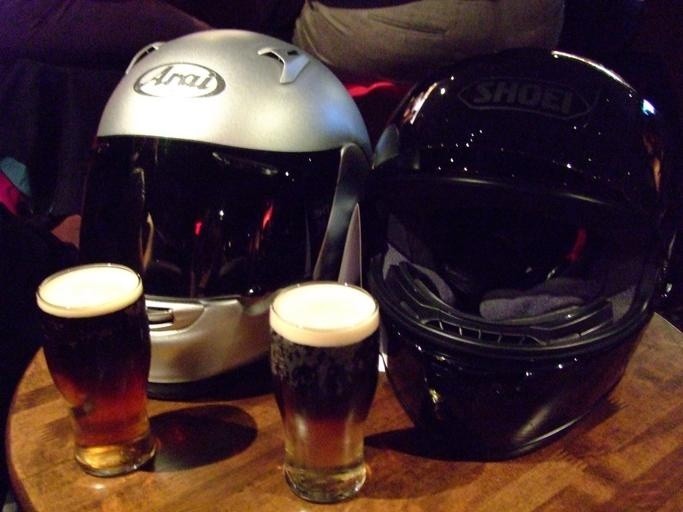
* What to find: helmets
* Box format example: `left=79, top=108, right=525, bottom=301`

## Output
left=372, top=47, right=674, bottom=462
left=78, top=28, right=371, bottom=400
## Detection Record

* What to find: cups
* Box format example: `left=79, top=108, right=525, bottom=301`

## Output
left=267, top=282, right=381, bottom=503
left=35, top=262, right=158, bottom=477
